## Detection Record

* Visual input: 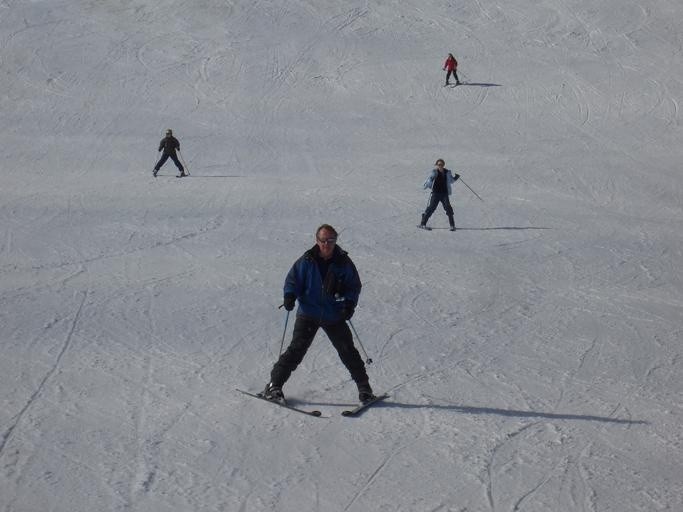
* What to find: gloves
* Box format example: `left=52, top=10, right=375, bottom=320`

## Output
left=343, top=299, right=355, bottom=320
left=283, top=292, right=297, bottom=312
left=453, top=173, right=460, bottom=181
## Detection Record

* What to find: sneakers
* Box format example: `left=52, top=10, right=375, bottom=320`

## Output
left=263, top=380, right=284, bottom=401
left=356, top=380, right=373, bottom=402
left=421, top=218, right=427, bottom=226
left=152, top=168, right=158, bottom=174
left=450, top=220, right=455, bottom=228
left=180, top=170, right=185, bottom=176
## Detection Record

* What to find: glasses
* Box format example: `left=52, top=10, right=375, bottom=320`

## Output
left=318, top=236, right=335, bottom=243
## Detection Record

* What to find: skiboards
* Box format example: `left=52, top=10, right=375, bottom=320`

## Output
left=153, top=173, right=190, bottom=177
left=236, top=389, right=390, bottom=416
left=444, top=83, right=462, bottom=88
left=417, top=225, right=456, bottom=231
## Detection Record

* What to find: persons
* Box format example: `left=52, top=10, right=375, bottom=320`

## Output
left=420, top=159, right=459, bottom=231
left=152, top=128, right=185, bottom=177
left=442, top=53, right=459, bottom=86
left=255, top=225, right=376, bottom=404
